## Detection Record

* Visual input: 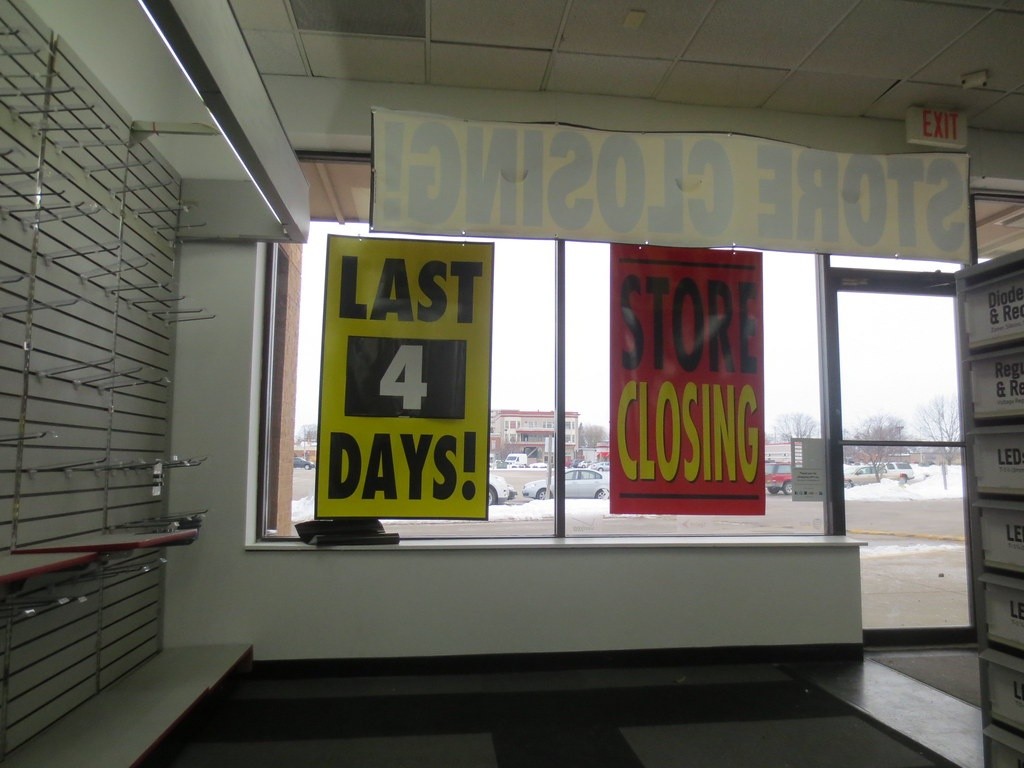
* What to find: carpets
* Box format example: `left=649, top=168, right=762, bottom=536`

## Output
left=866, top=647, right=981, bottom=710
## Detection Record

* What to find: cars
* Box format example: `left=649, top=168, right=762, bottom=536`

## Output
left=488, top=474, right=518, bottom=506
left=293, top=456, right=316, bottom=470
left=571, top=459, right=610, bottom=472
left=490, top=460, right=548, bottom=469
left=521, top=468, right=610, bottom=500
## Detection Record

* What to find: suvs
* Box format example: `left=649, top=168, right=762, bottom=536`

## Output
left=843, top=462, right=916, bottom=488
left=765, top=462, right=792, bottom=495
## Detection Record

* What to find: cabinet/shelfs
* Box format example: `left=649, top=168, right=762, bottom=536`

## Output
left=0, top=529, right=254, bottom=768
left=953, top=247, right=1024, bottom=768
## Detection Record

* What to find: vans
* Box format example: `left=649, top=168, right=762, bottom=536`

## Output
left=505, top=453, right=528, bottom=464
